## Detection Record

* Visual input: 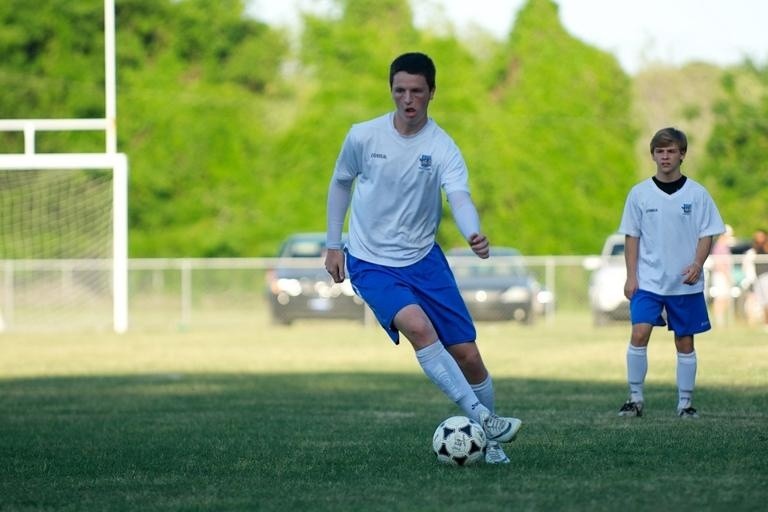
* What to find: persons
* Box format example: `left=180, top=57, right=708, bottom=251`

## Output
left=616, top=127, right=727, bottom=418
left=323, top=52, right=523, bottom=466
left=745, top=229, right=768, bottom=324
left=709, top=225, right=734, bottom=326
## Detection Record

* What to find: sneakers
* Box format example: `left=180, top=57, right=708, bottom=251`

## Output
left=616, top=398, right=644, bottom=418
left=676, top=402, right=703, bottom=419
left=478, top=410, right=524, bottom=444
left=483, top=439, right=511, bottom=465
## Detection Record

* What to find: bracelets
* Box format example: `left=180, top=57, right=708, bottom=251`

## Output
left=694, top=262, right=702, bottom=272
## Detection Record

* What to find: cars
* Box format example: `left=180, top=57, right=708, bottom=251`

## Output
left=258, top=233, right=367, bottom=327
left=444, top=246, right=537, bottom=325
left=584, top=233, right=630, bottom=326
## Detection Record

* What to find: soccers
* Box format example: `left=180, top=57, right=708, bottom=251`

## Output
left=433, top=416, right=487, bottom=468
